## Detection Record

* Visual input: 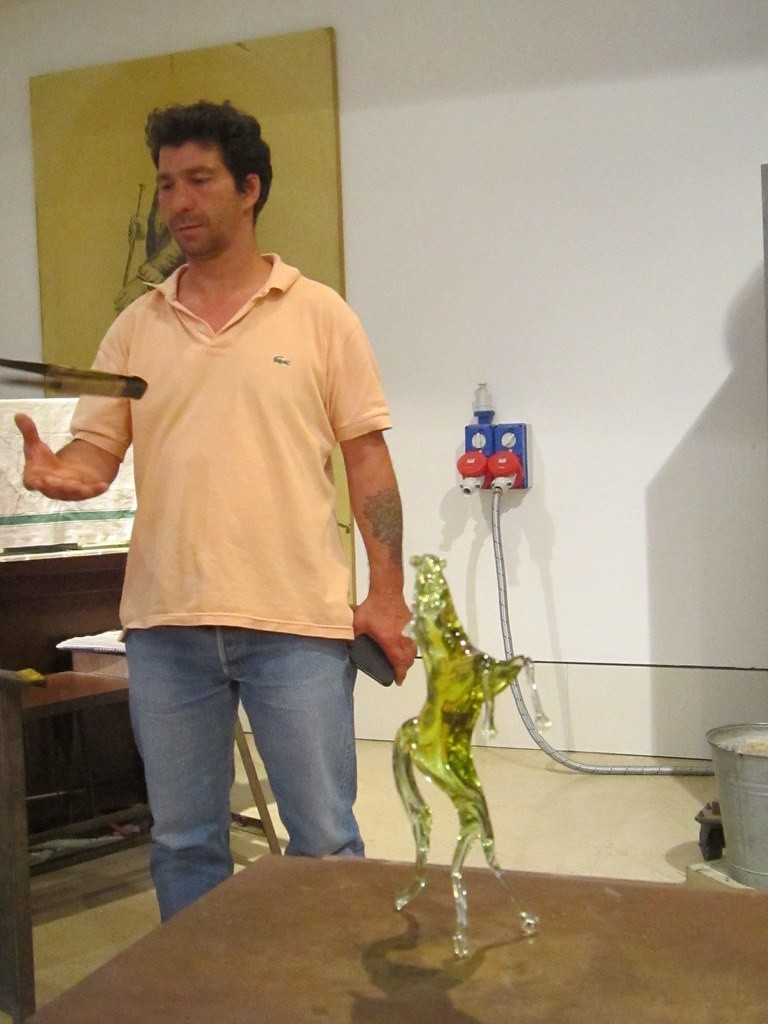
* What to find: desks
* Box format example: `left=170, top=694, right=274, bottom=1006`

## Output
left=1, top=553, right=153, bottom=875
left=21, top=854, right=768, bottom=1024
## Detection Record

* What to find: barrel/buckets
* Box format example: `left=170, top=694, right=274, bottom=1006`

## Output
left=703, top=721, right=768, bottom=890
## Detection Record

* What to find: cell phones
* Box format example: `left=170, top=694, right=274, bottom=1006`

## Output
left=349, top=634, right=395, bottom=687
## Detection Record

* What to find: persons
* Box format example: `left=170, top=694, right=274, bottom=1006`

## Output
left=12, top=96, right=419, bottom=928
